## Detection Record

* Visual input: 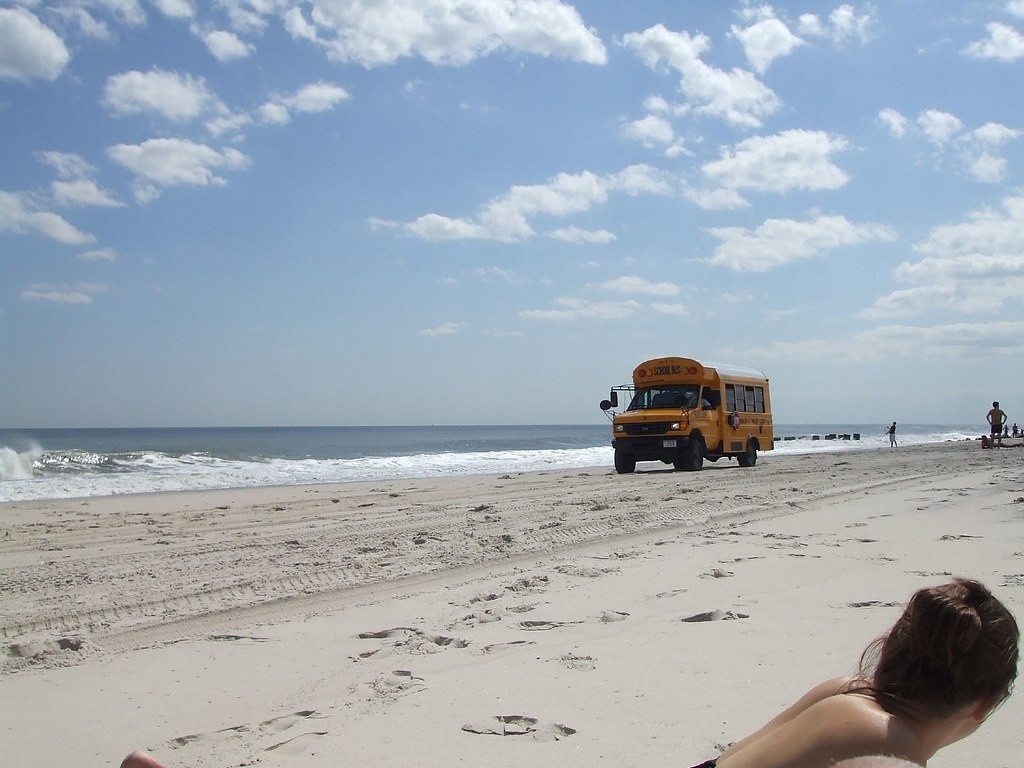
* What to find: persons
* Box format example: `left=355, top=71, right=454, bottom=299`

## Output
left=886, top=422, right=898, bottom=447
left=981, top=401, right=1024, bottom=449
left=689, top=391, right=712, bottom=409
left=691, top=577, right=1021, bottom=768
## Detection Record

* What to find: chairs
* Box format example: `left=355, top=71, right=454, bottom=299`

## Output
left=652, top=391, right=683, bottom=407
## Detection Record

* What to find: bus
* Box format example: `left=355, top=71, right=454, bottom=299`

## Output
left=600, top=357, right=774, bottom=474
